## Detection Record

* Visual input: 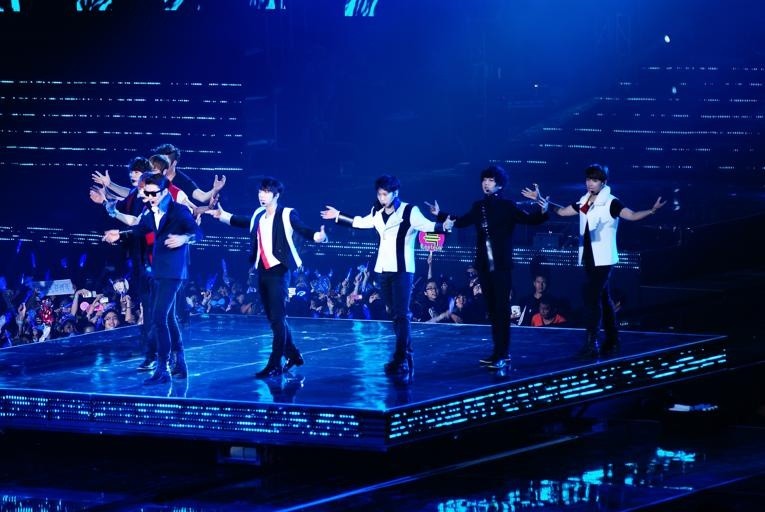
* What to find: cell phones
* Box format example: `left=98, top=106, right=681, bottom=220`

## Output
left=351, top=295, right=362, bottom=304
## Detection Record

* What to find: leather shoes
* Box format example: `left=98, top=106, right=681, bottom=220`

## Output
left=384, top=353, right=414, bottom=386
left=477, top=349, right=511, bottom=370
left=136, top=350, right=159, bottom=370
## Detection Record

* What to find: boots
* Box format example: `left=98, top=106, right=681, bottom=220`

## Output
left=573, top=332, right=600, bottom=359
left=170, top=350, right=188, bottom=379
left=144, top=360, right=172, bottom=386
left=600, top=329, right=622, bottom=355
left=282, top=344, right=304, bottom=373
left=255, top=353, right=282, bottom=379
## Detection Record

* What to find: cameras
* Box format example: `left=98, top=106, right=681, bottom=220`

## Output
left=83, top=291, right=96, bottom=298
left=512, top=306, right=520, bottom=317
left=99, top=297, right=108, bottom=304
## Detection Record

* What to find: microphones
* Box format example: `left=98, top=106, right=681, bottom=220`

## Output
left=591, top=185, right=603, bottom=194
left=262, top=195, right=277, bottom=205
left=132, top=179, right=138, bottom=182
left=383, top=195, right=394, bottom=206
left=151, top=191, right=166, bottom=204
left=486, top=186, right=500, bottom=192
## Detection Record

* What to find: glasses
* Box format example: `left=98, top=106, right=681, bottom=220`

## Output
left=143, top=188, right=165, bottom=197
